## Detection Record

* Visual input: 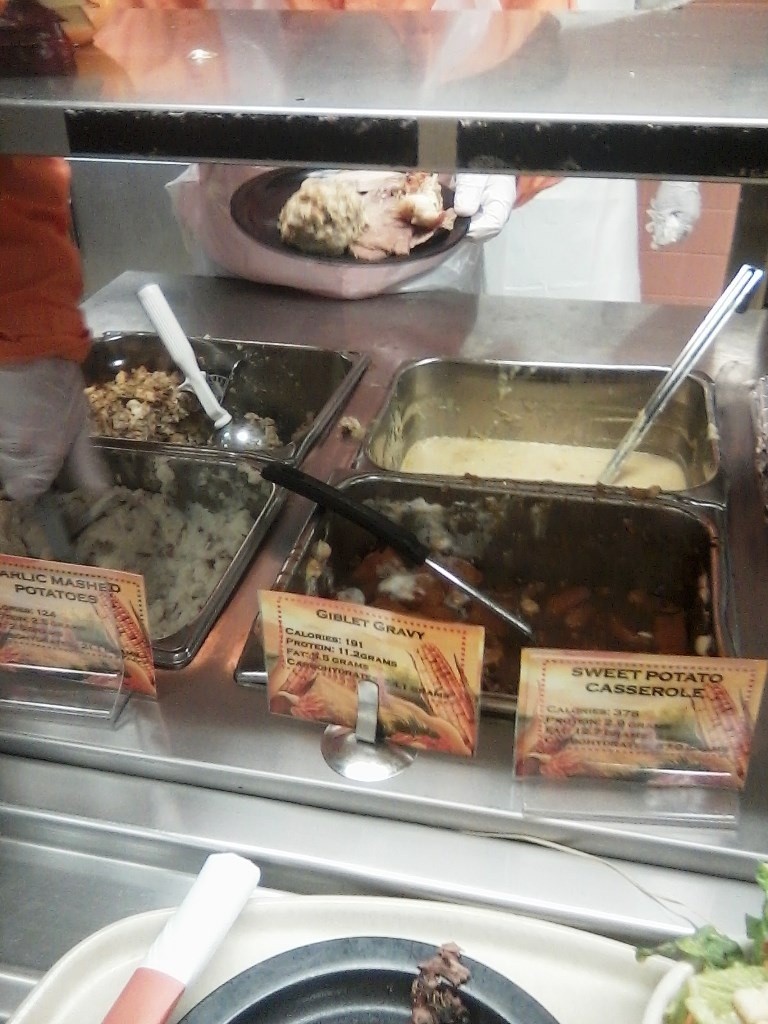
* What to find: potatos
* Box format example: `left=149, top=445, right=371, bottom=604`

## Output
left=323, top=548, right=690, bottom=697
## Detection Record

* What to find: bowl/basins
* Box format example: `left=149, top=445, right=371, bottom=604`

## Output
left=642, top=936, right=755, bottom=1024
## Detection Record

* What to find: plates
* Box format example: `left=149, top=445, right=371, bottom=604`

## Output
left=174, top=937, right=560, bottom=1024
left=231, top=167, right=472, bottom=265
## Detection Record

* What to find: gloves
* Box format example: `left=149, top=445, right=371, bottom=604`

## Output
left=0, top=356, right=112, bottom=500
left=449, top=173, right=517, bottom=242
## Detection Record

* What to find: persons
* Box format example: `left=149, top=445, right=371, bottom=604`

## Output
left=1, top=155, right=563, bottom=498
